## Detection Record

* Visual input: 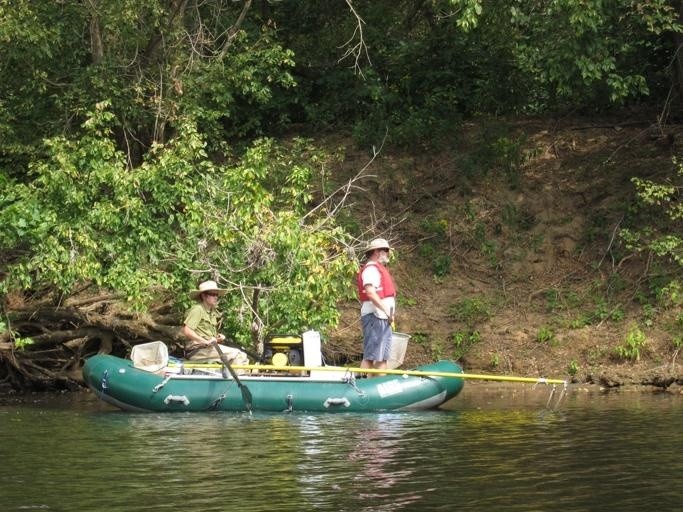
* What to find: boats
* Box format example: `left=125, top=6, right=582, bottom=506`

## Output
left=80, top=353, right=465, bottom=420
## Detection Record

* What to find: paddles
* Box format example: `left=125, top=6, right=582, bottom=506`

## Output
left=211, top=340, right=253, bottom=410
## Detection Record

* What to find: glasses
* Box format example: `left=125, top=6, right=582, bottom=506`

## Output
left=378, top=247, right=389, bottom=252
left=202, top=292, right=220, bottom=297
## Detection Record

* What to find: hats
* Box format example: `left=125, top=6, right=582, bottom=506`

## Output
left=190, top=281, right=228, bottom=299
left=363, top=239, right=390, bottom=254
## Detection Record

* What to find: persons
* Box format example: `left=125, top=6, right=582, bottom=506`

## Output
left=181, top=280, right=248, bottom=375
left=357, top=238, right=396, bottom=378
